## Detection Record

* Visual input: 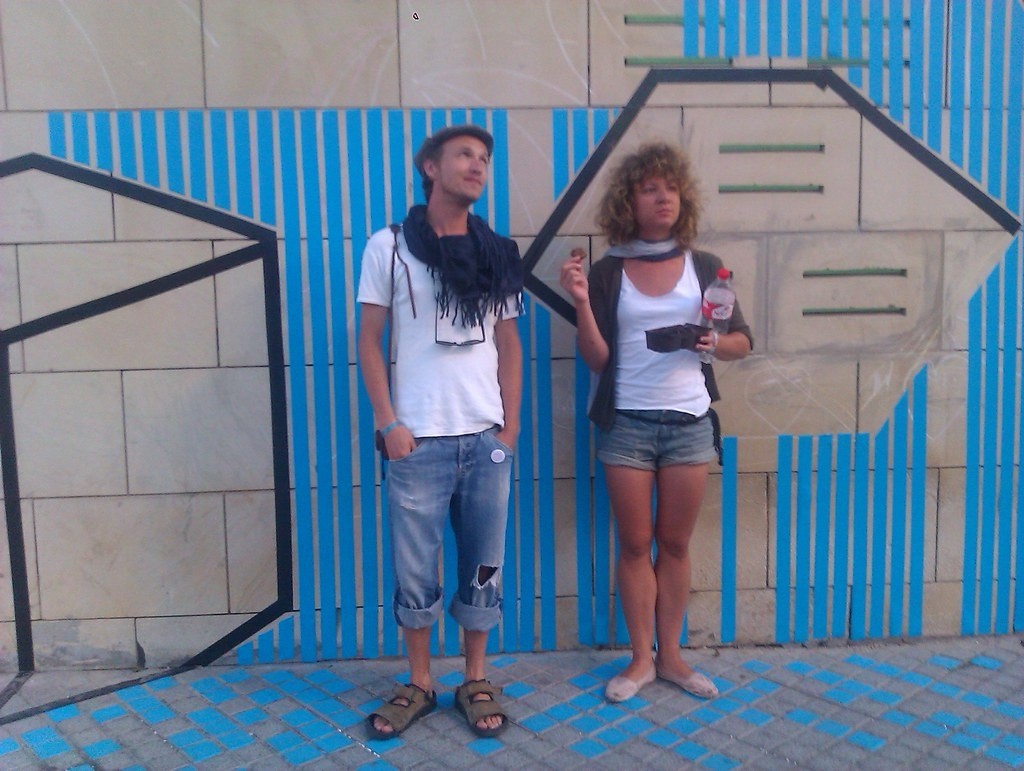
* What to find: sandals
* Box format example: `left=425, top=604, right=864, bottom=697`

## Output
left=454, top=679, right=508, bottom=737
left=365, top=682, right=437, bottom=741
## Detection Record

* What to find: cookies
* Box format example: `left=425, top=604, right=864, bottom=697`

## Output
left=571, top=247, right=587, bottom=260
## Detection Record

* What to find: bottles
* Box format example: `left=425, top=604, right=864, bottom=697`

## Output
left=698, top=268, right=736, bottom=364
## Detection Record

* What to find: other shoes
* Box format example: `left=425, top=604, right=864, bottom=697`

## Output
left=604, top=657, right=655, bottom=702
left=656, top=662, right=718, bottom=699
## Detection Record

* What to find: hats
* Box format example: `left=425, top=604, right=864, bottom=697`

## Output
left=414, top=125, right=494, bottom=177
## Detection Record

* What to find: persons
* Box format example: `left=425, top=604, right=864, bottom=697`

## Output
left=356, top=126, right=527, bottom=741
left=559, top=142, right=754, bottom=702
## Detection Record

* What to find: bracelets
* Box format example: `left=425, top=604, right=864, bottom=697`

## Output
left=710, top=331, right=719, bottom=354
left=382, top=421, right=402, bottom=437
